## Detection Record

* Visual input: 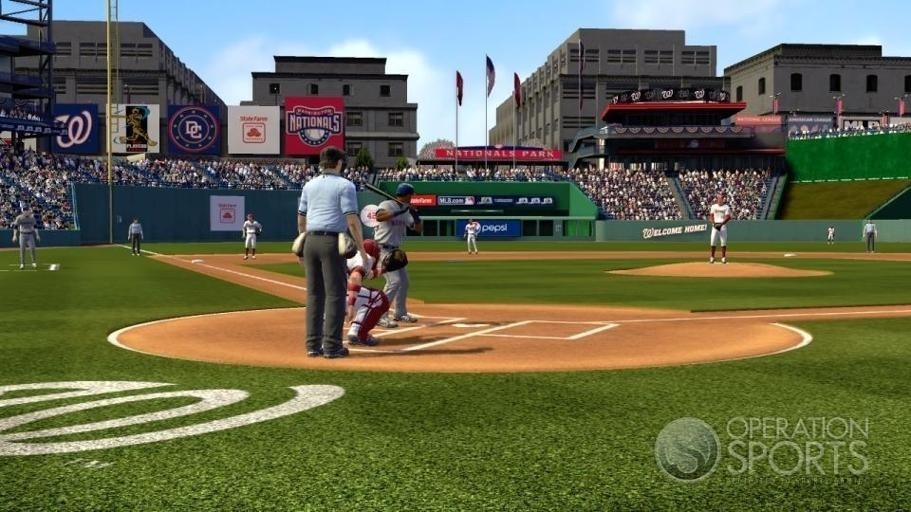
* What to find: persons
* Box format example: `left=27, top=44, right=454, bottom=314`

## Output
left=242, top=212, right=264, bottom=261
left=345, top=238, right=410, bottom=345
left=73, top=153, right=566, bottom=191
left=0, top=96, right=77, bottom=233
left=297, top=146, right=369, bottom=358
left=710, top=195, right=731, bottom=263
left=464, top=218, right=480, bottom=255
left=10, top=205, right=44, bottom=269
left=567, top=164, right=773, bottom=221
left=126, top=217, right=145, bottom=257
left=863, top=219, right=879, bottom=253
left=827, top=225, right=836, bottom=246
left=375, top=182, right=425, bottom=327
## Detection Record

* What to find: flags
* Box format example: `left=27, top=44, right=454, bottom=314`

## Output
left=485, top=55, right=497, bottom=98
left=514, top=72, right=522, bottom=111
left=457, top=71, right=465, bottom=107
